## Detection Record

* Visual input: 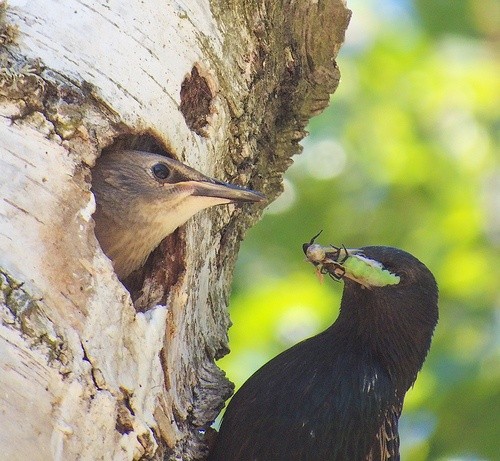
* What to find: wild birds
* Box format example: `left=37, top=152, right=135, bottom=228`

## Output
left=91, top=151, right=268, bottom=283
left=195, top=243, right=439, bottom=461
